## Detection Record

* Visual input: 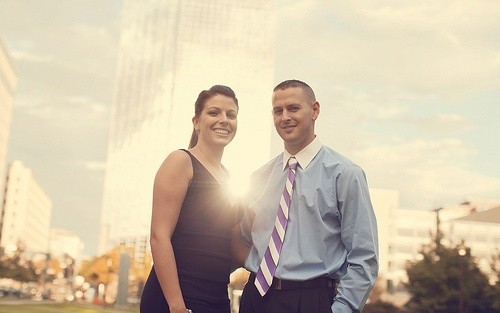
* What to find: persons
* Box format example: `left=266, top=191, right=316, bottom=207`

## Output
left=140, top=85, right=243, bottom=313
left=239, top=80, right=379, bottom=313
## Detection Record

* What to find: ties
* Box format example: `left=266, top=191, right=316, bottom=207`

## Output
left=254, top=157, right=297, bottom=297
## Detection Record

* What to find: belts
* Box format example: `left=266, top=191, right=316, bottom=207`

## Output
left=247, top=272, right=338, bottom=291
